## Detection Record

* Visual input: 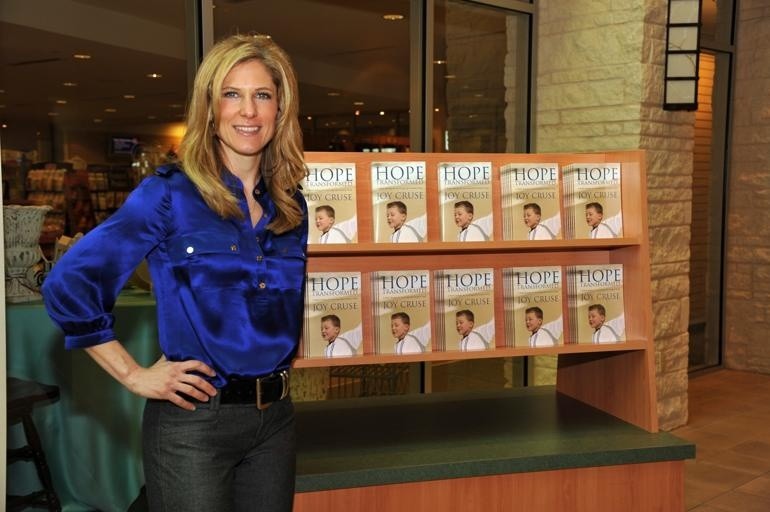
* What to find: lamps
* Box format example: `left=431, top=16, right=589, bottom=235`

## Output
left=664, top=0, right=702, bottom=112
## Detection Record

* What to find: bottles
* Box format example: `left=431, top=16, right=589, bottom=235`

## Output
left=25, top=165, right=129, bottom=244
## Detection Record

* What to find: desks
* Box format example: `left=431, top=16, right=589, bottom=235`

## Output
left=6, top=286, right=160, bottom=512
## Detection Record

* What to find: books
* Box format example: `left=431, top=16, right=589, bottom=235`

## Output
left=301, top=162, right=357, bottom=243
left=436, top=162, right=494, bottom=241
left=370, top=161, right=428, bottom=242
left=498, top=163, right=622, bottom=241
left=304, top=263, right=628, bottom=359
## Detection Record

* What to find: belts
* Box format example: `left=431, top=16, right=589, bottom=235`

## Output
left=176, top=370, right=289, bottom=409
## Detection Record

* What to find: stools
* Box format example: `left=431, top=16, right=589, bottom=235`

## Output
left=7, top=376, right=62, bottom=512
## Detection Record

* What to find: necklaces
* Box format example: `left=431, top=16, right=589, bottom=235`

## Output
left=247, top=197, right=258, bottom=212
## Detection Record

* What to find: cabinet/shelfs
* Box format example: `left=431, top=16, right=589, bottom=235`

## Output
left=2, top=160, right=141, bottom=258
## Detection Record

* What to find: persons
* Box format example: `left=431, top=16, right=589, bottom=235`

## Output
left=41, top=31, right=307, bottom=511
left=386, top=202, right=421, bottom=243
left=312, top=204, right=349, bottom=244
left=452, top=202, right=485, bottom=240
